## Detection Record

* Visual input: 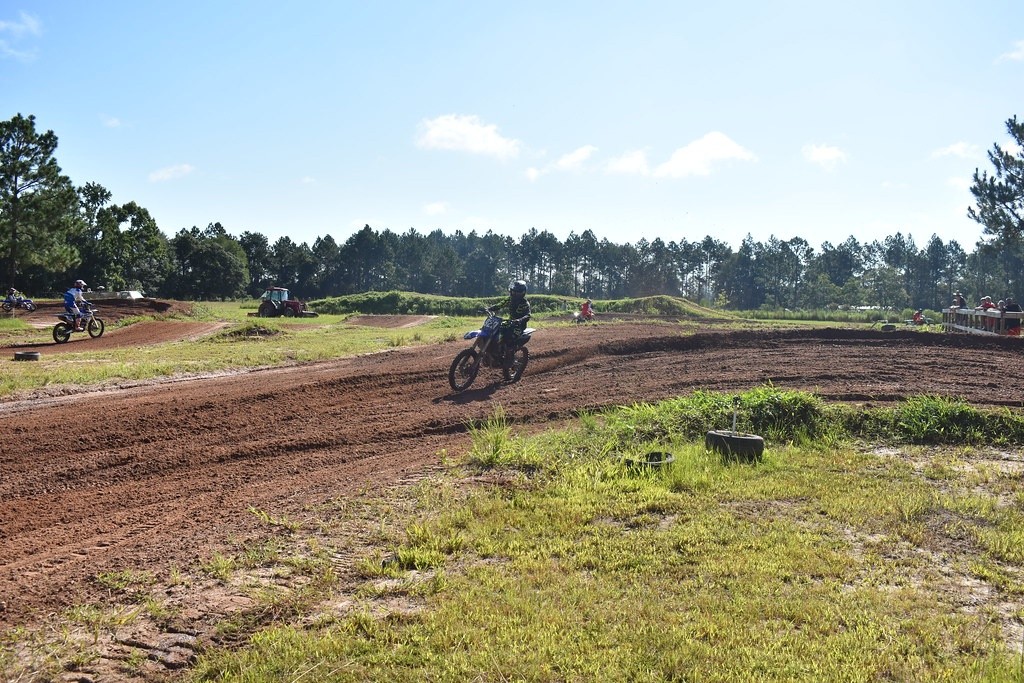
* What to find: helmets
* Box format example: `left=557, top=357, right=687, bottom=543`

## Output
left=73, top=280, right=87, bottom=290
left=588, top=299, right=592, bottom=303
left=10, top=288, right=15, bottom=294
left=508, top=280, right=528, bottom=300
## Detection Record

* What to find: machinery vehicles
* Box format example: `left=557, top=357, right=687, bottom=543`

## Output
left=246, top=286, right=319, bottom=319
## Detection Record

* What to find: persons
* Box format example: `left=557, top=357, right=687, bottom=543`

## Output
left=912, top=308, right=924, bottom=323
left=8, top=288, right=24, bottom=308
left=581, top=299, right=595, bottom=320
left=950, top=290, right=1023, bottom=336
left=65, top=279, right=88, bottom=331
left=477, top=280, right=530, bottom=380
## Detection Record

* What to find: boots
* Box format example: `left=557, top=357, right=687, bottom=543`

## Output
left=75, top=318, right=83, bottom=330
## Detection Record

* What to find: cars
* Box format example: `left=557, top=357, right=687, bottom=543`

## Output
left=117, top=290, right=145, bottom=299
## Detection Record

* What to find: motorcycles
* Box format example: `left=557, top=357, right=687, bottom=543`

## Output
left=51, top=303, right=104, bottom=344
left=447, top=307, right=536, bottom=392
left=0, top=292, right=37, bottom=313
left=571, top=308, right=596, bottom=324
